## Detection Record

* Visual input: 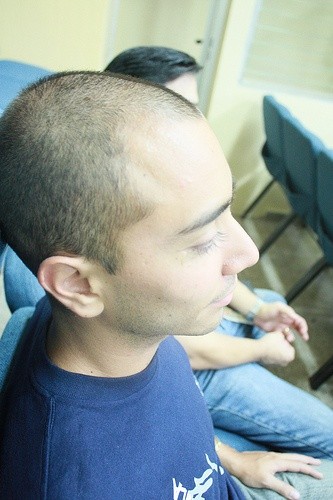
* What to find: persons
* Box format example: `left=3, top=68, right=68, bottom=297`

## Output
left=0, top=71, right=333, bottom=500
left=103, top=46, right=332, bottom=459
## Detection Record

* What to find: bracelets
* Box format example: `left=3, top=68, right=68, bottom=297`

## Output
left=245, top=300, right=264, bottom=322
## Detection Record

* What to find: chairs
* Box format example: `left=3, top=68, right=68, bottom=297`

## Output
left=0, top=58, right=56, bottom=115
left=240, top=96, right=333, bottom=390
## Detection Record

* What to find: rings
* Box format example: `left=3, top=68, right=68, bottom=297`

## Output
left=282, top=327, right=289, bottom=334
